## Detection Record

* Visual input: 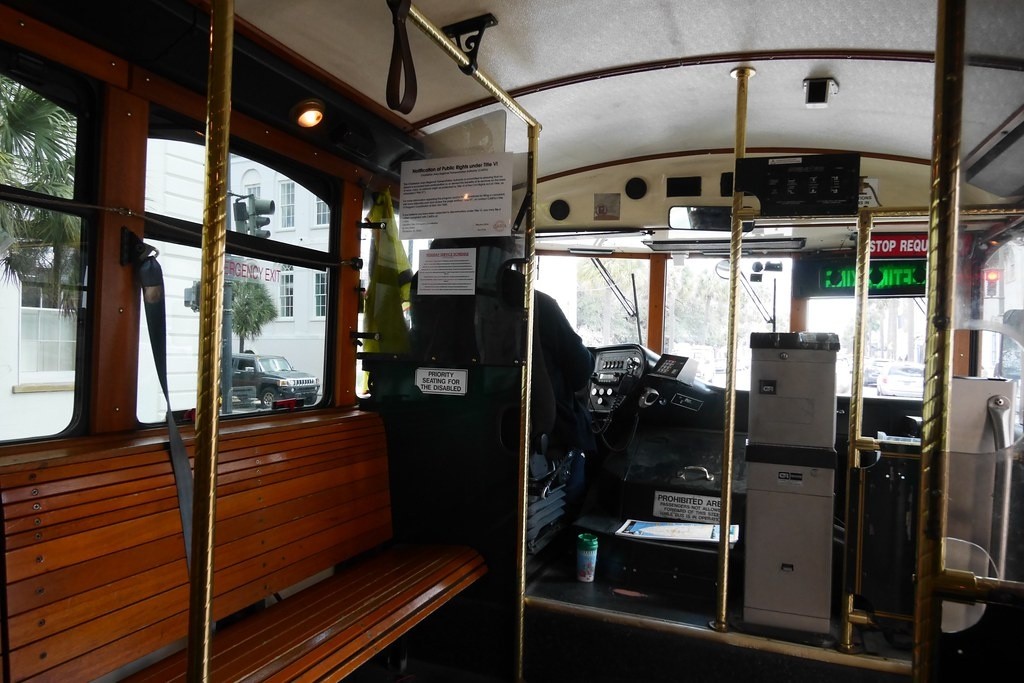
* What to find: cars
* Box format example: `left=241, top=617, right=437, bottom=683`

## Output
left=671, top=342, right=752, bottom=384
left=863, top=357, right=886, bottom=387
left=877, top=360, right=925, bottom=399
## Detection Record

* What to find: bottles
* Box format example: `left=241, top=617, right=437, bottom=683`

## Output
left=578, top=533, right=598, bottom=582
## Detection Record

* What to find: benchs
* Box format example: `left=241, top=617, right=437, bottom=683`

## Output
left=0, top=406, right=488, bottom=683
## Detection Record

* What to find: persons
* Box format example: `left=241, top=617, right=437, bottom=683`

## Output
left=511, top=236, right=602, bottom=496
left=688, top=210, right=712, bottom=230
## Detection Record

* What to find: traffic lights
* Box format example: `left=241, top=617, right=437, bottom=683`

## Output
left=248, top=194, right=276, bottom=239
left=987, top=269, right=997, bottom=297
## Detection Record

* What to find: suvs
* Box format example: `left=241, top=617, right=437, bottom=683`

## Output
left=231, top=353, right=321, bottom=409
